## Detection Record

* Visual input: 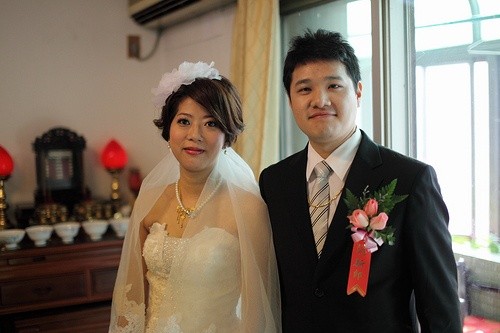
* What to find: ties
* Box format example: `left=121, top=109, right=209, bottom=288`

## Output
left=309, top=159, right=333, bottom=259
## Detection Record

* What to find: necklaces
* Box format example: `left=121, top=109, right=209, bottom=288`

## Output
left=174, top=173, right=225, bottom=228
left=306, top=179, right=346, bottom=209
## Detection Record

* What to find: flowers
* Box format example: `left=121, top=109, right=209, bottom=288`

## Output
left=152, top=59, right=222, bottom=111
left=343, top=178, right=409, bottom=253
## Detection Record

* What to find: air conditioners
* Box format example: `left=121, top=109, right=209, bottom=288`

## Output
left=128, top=0, right=237, bottom=31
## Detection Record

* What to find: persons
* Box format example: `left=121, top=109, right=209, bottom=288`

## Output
left=108, top=61, right=282, bottom=332
left=260, top=29, right=464, bottom=333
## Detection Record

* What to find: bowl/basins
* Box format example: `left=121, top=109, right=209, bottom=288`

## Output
left=26, top=225, right=54, bottom=247
left=0, top=229, right=26, bottom=249
left=81, top=221, right=109, bottom=240
left=54, top=222, right=80, bottom=243
left=108, top=219, right=132, bottom=238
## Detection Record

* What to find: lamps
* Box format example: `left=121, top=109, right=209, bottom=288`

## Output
left=100, top=139, right=127, bottom=201
left=0, top=146, right=15, bottom=228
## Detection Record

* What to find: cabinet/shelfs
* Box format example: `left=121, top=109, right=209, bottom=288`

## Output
left=0, top=239, right=124, bottom=333
